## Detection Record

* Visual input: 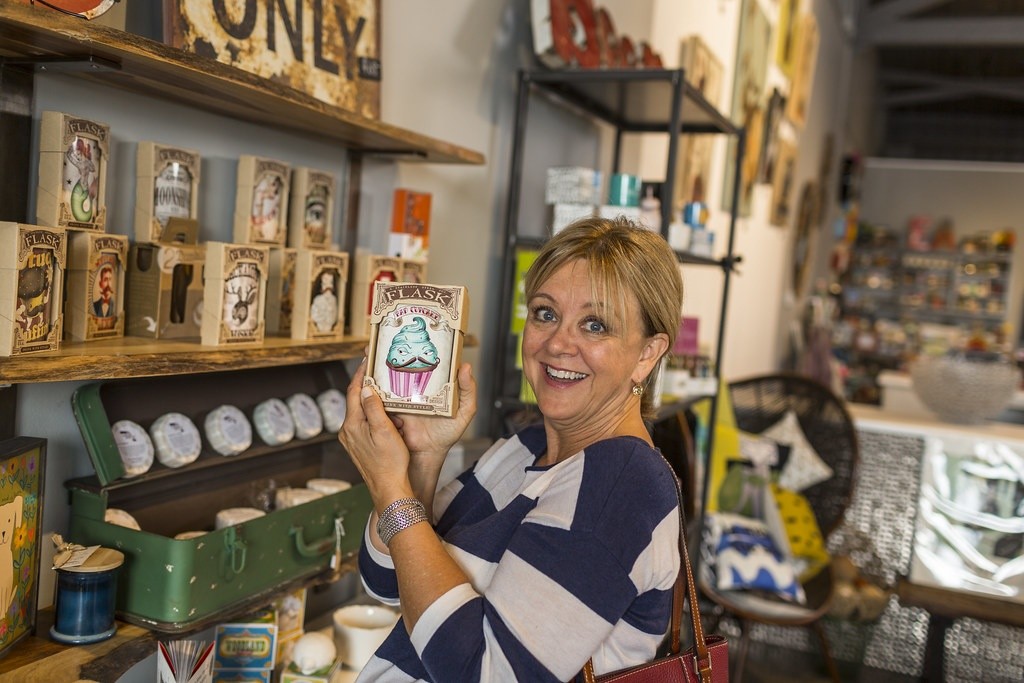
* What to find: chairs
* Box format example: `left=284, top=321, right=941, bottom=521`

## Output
left=693, top=377, right=859, bottom=683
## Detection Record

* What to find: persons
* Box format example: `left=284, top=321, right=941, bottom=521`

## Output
left=339, top=216, right=680, bottom=683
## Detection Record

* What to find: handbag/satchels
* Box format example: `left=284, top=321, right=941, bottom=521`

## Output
left=575, top=437, right=729, bottom=683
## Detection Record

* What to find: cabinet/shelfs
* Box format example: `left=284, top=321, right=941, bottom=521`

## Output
left=487, top=67, right=748, bottom=648
left=0, top=0, right=494, bottom=683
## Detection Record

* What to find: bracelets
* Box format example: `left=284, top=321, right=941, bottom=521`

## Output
left=376, top=498, right=428, bottom=545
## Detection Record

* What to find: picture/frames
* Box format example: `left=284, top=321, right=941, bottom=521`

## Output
left=666, top=32, right=731, bottom=268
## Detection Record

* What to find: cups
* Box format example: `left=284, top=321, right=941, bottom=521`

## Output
left=335, top=605, right=401, bottom=672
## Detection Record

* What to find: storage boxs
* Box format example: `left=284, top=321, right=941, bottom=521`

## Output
left=0, top=111, right=470, bottom=683
